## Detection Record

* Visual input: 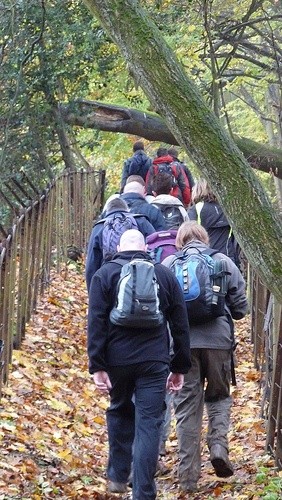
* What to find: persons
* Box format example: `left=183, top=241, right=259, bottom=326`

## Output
left=159, top=220, right=248, bottom=490
left=87, top=228, right=191, bottom=500
left=86, top=197, right=157, bottom=295
left=108, top=148, right=195, bottom=230
left=187, top=178, right=241, bottom=271
left=121, top=142, right=152, bottom=192
left=146, top=214, right=185, bottom=454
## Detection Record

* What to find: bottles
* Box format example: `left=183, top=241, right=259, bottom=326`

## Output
left=211, top=285, right=220, bottom=305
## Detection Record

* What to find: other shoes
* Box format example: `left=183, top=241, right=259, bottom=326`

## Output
left=109, top=477, right=127, bottom=493
left=210, top=444, right=234, bottom=477
left=182, top=479, right=197, bottom=492
left=159, top=441, right=168, bottom=455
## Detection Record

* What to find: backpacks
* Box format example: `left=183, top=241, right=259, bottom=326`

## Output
left=168, top=249, right=231, bottom=317
left=151, top=161, right=180, bottom=187
left=155, top=203, right=185, bottom=230
left=93, top=213, right=152, bottom=261
left=108, top=258, right=164, bottom=328
left=128, top=157, right=149, bottom=180
